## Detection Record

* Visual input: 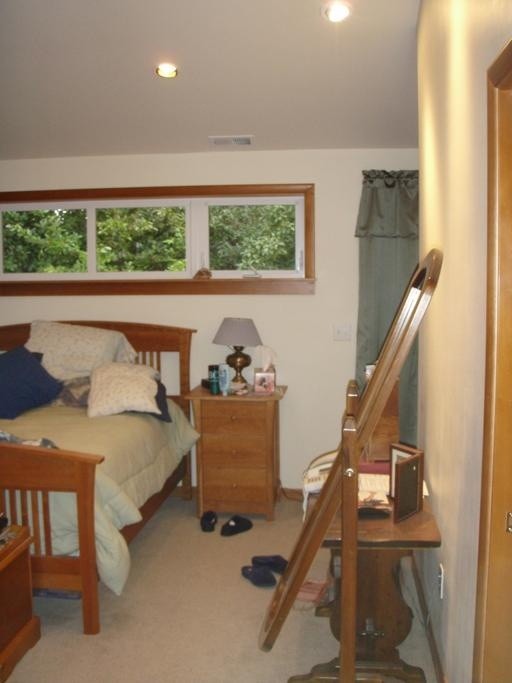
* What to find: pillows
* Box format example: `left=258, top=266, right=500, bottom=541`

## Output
left=1, top=321, right=171, bottom=424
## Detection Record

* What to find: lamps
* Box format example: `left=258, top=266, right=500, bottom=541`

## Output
left=211, top=316, right=264, bottom=395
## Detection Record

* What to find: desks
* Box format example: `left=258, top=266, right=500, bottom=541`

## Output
left=287, top=492, right=441, bottom=682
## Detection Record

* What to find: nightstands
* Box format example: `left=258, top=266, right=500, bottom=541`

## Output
left=192, top=397, right=281, bottom=519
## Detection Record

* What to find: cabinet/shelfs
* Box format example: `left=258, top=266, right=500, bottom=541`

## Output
left=1, top=525, right=41, bottom=682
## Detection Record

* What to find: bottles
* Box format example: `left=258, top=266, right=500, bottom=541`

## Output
left=219, top=364, right=229, bottom=397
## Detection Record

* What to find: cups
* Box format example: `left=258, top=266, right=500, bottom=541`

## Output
left=208, top=364, right=220, bottom=394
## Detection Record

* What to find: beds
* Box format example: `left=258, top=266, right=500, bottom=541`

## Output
left=0, top=321, right=197, bottom=635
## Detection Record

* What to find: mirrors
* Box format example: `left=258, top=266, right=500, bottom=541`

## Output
left=257, top=249, right=445, bottom=680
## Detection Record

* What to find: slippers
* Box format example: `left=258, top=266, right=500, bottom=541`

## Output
left=200, top=511, right=252, bottom=535
left=242, top=556, right=288, bottom=585
